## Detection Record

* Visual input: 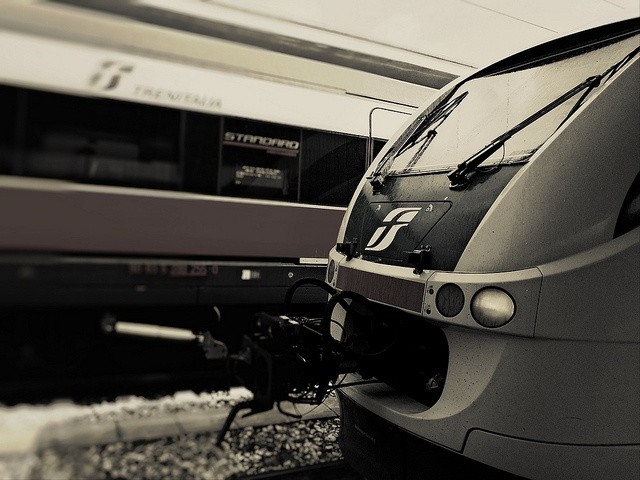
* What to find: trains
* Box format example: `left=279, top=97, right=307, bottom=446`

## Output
left=1, top=1, right=458, bottom=317
left=323, top=16, right=639, bottom=479
